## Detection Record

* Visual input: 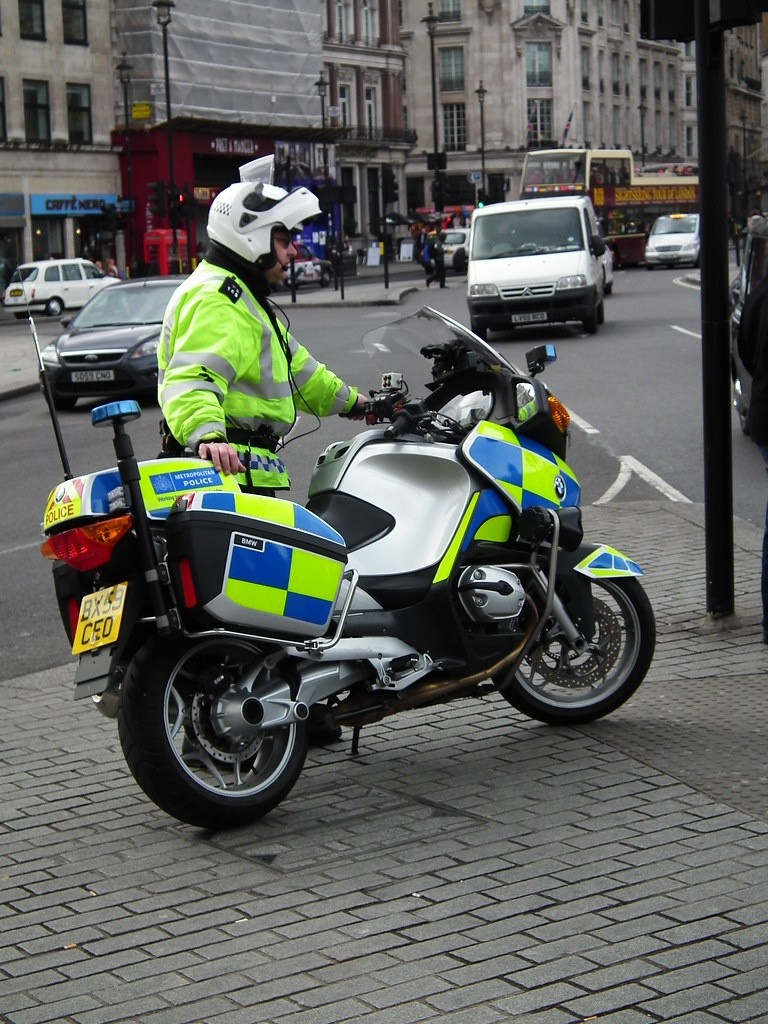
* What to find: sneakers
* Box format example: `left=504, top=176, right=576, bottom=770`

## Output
left=306, top=720, right=341, bottom=746
left=182, top=730, right=256, bottom=773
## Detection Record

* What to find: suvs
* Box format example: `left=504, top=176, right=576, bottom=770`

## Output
left=2, top=258, right=122, bottom=318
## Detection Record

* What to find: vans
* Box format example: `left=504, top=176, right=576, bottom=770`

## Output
left=645, top=213, right=700, bottom=269
left=467, top=195, right=607, bottom=342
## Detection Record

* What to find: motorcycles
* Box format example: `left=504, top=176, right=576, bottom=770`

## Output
left=27, top=305, right=656, bottom=834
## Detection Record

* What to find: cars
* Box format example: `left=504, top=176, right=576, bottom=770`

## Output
left=730, top=217, right=766, bottom=435
left=272, top=243, right=333, bottom=290
left=38, top=274, right=190, bottom=411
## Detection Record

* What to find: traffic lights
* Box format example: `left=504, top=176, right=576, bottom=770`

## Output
left=175, top=188, right=187, bottom=213
left=384, top=172, right=400, bottom=203
left=430, top=171, right=450, bottom=204
left=148, top=181, right=164, bottom=218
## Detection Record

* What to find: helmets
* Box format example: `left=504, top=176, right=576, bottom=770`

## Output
left=207, top=181, right=322, bottom=270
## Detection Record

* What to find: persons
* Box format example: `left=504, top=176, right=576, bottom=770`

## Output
left=727, top=210, right=764, bottom=250
left=157, top=182, right=375, bottom=772
left=95, top=258, right=119, bottom=277
left=738, top=276, right=768, bottom=646
left=531, top=160, right=696, bottom=186
left=341, top=221, right=467, bottom=289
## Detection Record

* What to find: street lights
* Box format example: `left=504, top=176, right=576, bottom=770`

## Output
left=474, top=79, right=488, bottom=196
left=152, top=0, right=176, bottom=278
left=423, top=12, right=442, bottom=172
left=115, top=52, right=133, bottom=268
left=313, top=73, right=331, bottom=259
left=738, top=109, right=748, bottom=217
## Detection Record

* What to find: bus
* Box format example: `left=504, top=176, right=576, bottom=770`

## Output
left=518, top=148, right=698, bottom=269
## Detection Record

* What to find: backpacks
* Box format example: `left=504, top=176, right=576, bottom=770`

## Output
left=428, top=242, right=438, bottom=259
